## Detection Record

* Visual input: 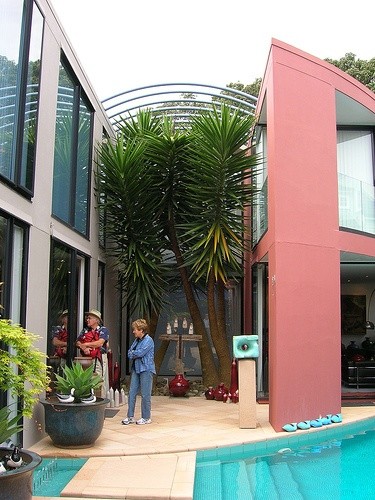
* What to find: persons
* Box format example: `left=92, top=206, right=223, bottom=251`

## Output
left=122, top=319, right=156, bottom=425
left=75, top=309, right=110, bottom=391
left=52, top=308, right=68, bottom=368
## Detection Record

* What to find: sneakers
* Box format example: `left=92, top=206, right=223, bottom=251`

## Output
left=136, top=418, right=151, bottom=425
left=122, top=417, right=135, bottom=425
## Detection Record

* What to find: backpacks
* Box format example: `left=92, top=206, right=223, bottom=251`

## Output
left=56, top=329, right=68, bottom=357
left=78, top=330, right=102, bottom=361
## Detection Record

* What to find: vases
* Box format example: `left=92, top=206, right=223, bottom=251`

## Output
left=205, top=387, right=215, bottom=400
left=362, top=337, right=373, bottom=349
left=169, top=374, right=189, bottom=397
left=214, top=384, right=228, bottom=401
left=347, top=341, right=359, bottom=350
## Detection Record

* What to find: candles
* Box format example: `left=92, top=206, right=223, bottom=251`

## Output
left=109, top=387, right=114, bottom=400
left=121, top=390, right=125, bottom=404
left=120, top=387, right=123, bottom=394
left=106, top=402, right=110, bottom=408
left=223, top=393, right=228, bottom=403
left=106, top=390, right=110, bottom=399
left=232, top=393, right=237, bottom=403
left=125, top=395, right=128, bottom=403
left=114, top=389, right=120, bottom=407
left=227, top=393, right=232, bottom=400
left=110, top=399, right=114, bottom=408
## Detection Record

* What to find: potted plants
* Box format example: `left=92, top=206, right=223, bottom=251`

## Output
left=0, top=315, right=53, bottom=500
left=39, top=359, right=110, bottom=450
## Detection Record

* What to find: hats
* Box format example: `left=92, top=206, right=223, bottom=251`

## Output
left=85, top=309, right=103, bottom=324
left=59, top=309, right=68, bottom=319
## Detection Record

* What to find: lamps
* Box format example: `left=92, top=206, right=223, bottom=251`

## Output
left=362, top=288, right=375, bottom=330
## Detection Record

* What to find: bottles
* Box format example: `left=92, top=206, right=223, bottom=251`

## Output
left=10, top=447, right=20, bottom=470
